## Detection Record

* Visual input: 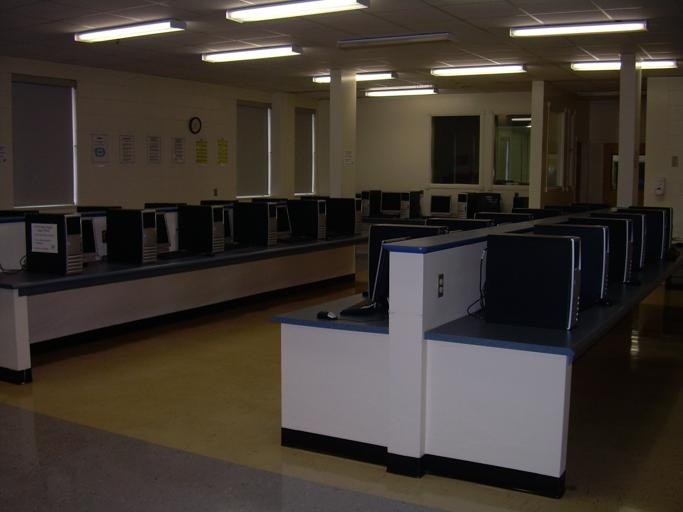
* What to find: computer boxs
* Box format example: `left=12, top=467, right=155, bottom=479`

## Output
left=104, top=208, right=158, bottom=263
left=368, top=224, right=449, bottom=300
left=456, top=193, right=467, bottom=218
left=425, top=218, right=496, bottom=234
left=512, top=203, right=673, bottom=306
left=485, top=233, right=583, bottom=331
left=287, top=199, right=327, bottom=240
left=361, top=190, right=381, bottom=218
left=23, top=212, right=84, bottom=275
left=474, top=212, right=534, bottom=226
left=237, top=201, right=278, bottom=248
left=400, top=191, right=419, bottom=219
left=177, top=205, right=225, bottom=254
left=333, top=197, right=363, bottom=234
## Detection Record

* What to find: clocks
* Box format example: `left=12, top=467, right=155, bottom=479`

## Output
left=189, top=117, right=201, bottom=134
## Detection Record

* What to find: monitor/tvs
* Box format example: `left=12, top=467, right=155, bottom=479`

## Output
left=426, top=190, right=455, bottom=218
left=75, top=205, right=122, bottom=265
left=368, top=235, right=418, bottom=321
left=252, top=197, right=292, bottom=241
left=200, top=200, right=240, bottom=249
left=300, top=194, right=331, bottom=237
left=467, top=193, right=500, bottom=219
left=144, top=202, right=188, bottom=257
left=380, top=191, right=400, bottom=218
left=0, top=209, right=40, bottom=274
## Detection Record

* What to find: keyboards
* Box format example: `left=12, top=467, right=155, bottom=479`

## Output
left=340, top=299, right=384, bottom=316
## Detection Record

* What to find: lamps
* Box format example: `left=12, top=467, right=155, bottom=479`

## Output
left=223, top=0, right=371, bottom=25
left=337, top=32, right=456, bottom=48
left=509, top=20, right=649, bottom=39
left=363, top=83, right=439, bottom=98
left=310, top=71, right=399, bottom=84
left=201, top=43, right=304, bottom=64
left=72, top=19, right=187, bottom=48
left=429, top=64, right=529, bottom=78
left=569, top=59, right=620, bottom=72
left=634, top=58, right=680, bottom=71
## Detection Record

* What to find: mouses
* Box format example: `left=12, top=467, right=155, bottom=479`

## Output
left=676, top=243, right=683, bottom=247
left=318, top=311, right=337, bottom=320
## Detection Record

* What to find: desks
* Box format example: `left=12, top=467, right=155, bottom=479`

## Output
left=2, top=190, right=506, bottom=385
left=266, top=207, right=682, bottom=499
